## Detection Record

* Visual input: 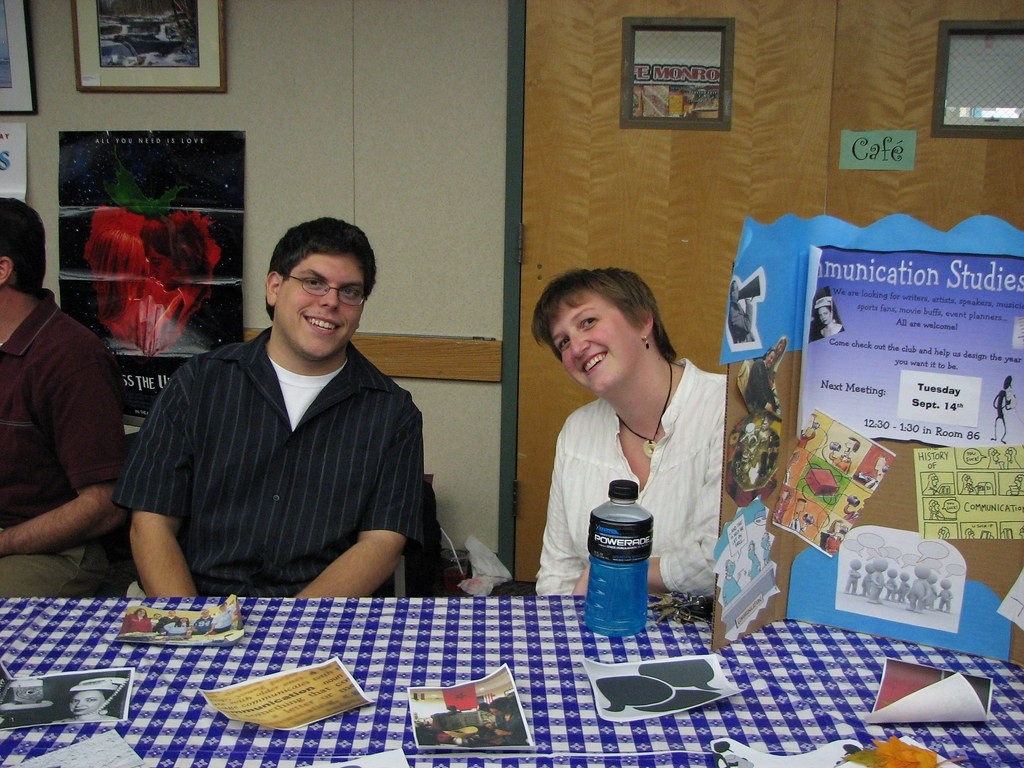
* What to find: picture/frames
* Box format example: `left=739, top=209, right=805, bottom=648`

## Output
left=72, top=0, right=228, bottom=94
left=0, top=0, right=39, bottom=116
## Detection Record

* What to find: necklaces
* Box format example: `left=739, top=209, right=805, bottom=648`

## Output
left=615, top=361, right=672, bottom=458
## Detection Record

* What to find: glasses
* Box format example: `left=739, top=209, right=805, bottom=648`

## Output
left=286, top=273, right=366, bottom=306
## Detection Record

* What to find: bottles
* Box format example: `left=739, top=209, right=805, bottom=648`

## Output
left=584, top=479, right=654, bottom=636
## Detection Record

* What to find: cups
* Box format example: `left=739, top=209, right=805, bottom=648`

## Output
left=440, top=549, right=470, bottom=593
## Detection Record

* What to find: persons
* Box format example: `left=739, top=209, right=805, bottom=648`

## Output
left=815, top=296, right=844, bottom=340
left=415, top=697, right=528, bottom=747
left=93, top=213, right=217, bottom=353
left=54, top=689, right=119, bottom=722
left=728, top=281, right=753, bottom=343
left=121, top=600, right=243, bottom=636
left=532, top=268, right=728, bottom=599
left=743, top=338, right=787, bottom=412
left=116, top=216, right=423, bottom=598
left=0, top=198, right=129, bottom=597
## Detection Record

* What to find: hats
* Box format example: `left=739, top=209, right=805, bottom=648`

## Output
left=69, top=679, right=118, bottom=691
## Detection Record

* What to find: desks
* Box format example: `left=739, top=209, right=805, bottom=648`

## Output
left=0, top=594, right=1024, bottom=768
